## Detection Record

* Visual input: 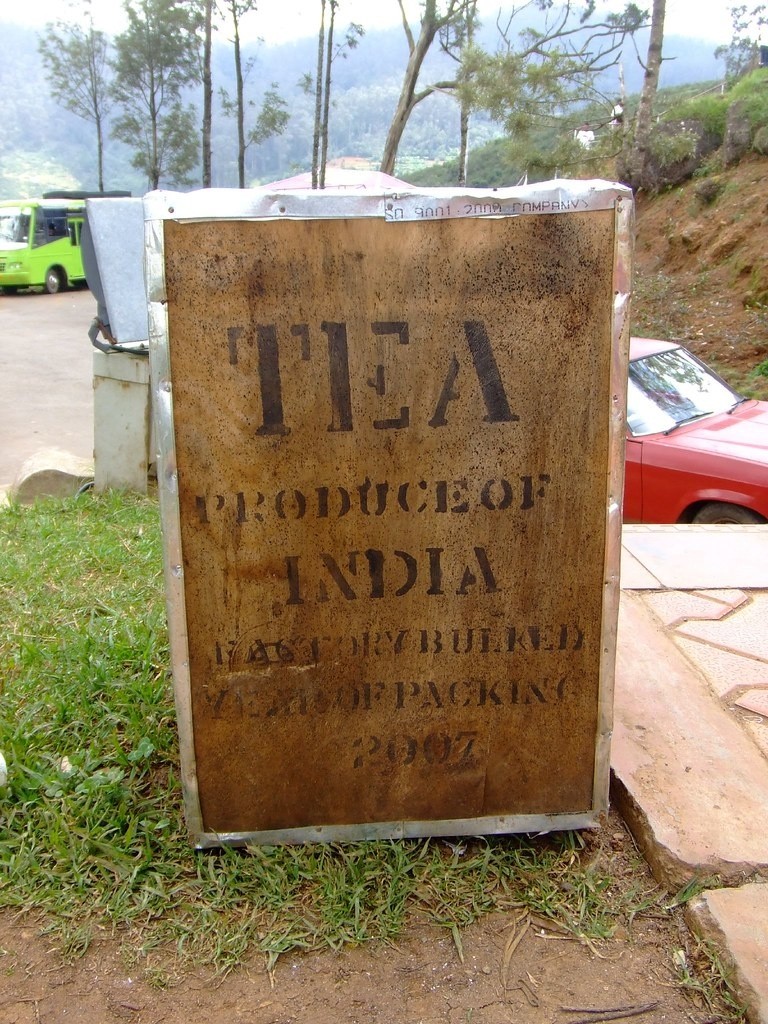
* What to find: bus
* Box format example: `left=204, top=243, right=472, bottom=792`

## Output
left=0, top=199, right=92, bottom=292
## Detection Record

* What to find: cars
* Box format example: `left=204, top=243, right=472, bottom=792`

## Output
left=625, top=338, right=768, bottom=525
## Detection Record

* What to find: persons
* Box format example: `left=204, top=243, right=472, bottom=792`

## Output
left=576, top=122, right=596, bottom=150
left=611, top=98, right=625, bottom=129
left=42, top=224, right=58, bottom=245
